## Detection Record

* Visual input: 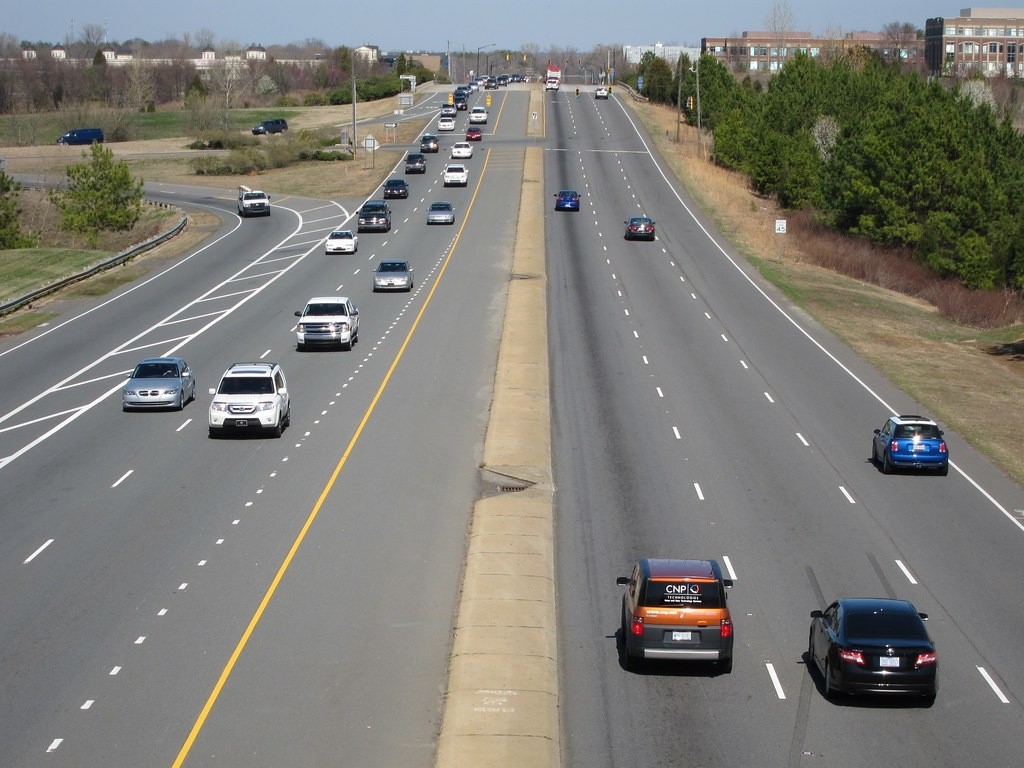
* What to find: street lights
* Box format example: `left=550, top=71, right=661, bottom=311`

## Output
left=689, top=58, right=703, bottom=158
left=476, top=43, right=496, bottom=78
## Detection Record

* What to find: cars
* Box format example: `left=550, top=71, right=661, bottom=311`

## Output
left=871, top=413, right=956, bottom=474
left=464, top=126, right=484, bottom=142
left=426, top=201, right=455, bottom=224
left=383, top=179, right=409, bottom=200
left=439, top=101, right=458, bottom=118
left=468, top=106, right=488, bottom=124
left=122, top=358, right=197, bottom=412
left=372, top=258, right=415, bottom=292
left=443, top=164, right=470, bottom=187
left=595, top=87, right=608, bottom=99
left=450, top=141, right=474, bottom=159
left=809, top=597, right=943, bottom=709
left=325, top=230, right=360, bottom=254
left=623, top=215, right=656, bottom=242
left=437, top=117, right=457, bottom=132
left=452, top=73, right=528, bottom=111
left=405, top=152, right=427, bottom=174
left=419, top=134, right=440, bottom=153
left=554, top=190, right=585, bottom=212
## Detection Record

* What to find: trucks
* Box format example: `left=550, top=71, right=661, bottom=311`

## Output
left=546, top=77, right=559, bottom=92
left=543, top=67, right=561, bottom=83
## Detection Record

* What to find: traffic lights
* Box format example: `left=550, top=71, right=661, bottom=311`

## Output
left=687, top=96, right=693, bottom=111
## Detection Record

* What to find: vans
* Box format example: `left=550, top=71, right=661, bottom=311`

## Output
left=55, top=128, right=105, bottom=147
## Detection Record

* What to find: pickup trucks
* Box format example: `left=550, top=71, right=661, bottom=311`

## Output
left=235, top=185, right=272, bottom=215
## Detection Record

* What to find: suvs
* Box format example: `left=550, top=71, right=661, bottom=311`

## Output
left=208, top=362, right=292, bottom=439
left=251, top=119, right=288, bottom=137
left=294, top=296, right=361, bottom=352
left=355, top=199, right=393, bottom=233
left=615, top=558, right=746, bottom=676
